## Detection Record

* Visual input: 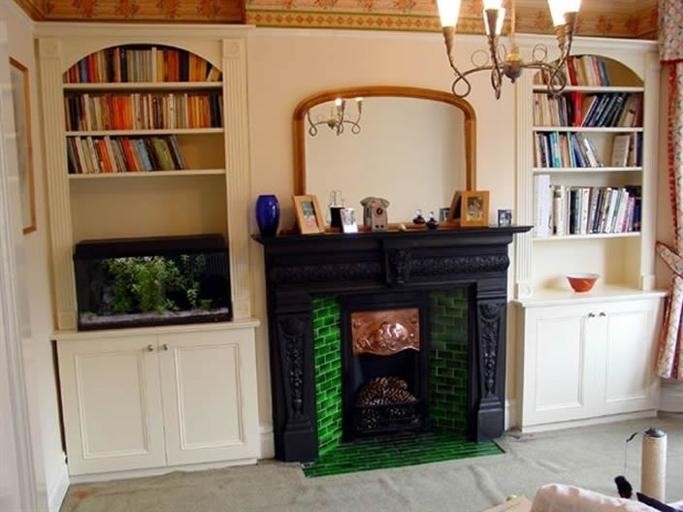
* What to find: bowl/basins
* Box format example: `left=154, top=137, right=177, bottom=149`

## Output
left=566, top=271, right=599, bottom=292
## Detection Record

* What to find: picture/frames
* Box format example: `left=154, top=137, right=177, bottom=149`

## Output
left=447, top=190, right=461, bottom=224
left=292, top=193, right=326, bottom=235
left=459, top=190, right=491, bottom=228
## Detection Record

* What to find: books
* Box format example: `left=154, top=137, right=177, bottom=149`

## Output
left=62, top=47, right=222, bottom=174
left=532, top=55, right=642, bottom=236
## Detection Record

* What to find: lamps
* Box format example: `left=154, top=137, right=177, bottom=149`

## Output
left=306, top=94, right=365, bottom=139
left=435, top=0, right=587, bottom=101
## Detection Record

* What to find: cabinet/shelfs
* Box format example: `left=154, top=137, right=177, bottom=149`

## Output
left=520, top=296, right=664, bottom=427
left=49, top=321, right=261, bottom=479
left=528, top=84, right=647, bottom=245
left=56, top=77, right=229, bottom=180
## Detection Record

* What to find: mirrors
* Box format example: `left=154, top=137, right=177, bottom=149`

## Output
left=288, top=83, right=481, bottom=230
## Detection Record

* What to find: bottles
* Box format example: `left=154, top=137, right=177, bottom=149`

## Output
left=414, top=210, right=425, bottom=225
left=425, top=212, right=439, bottom=229
